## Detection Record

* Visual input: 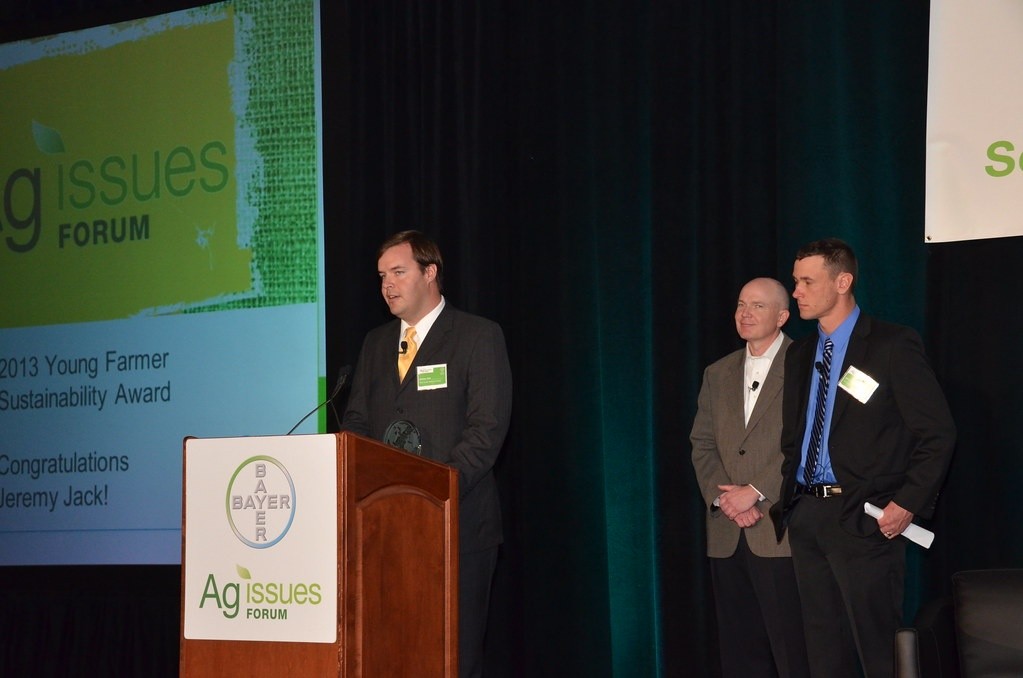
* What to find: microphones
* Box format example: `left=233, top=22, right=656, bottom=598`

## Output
left=401, top=341, right=408, bottom=354
left=287, top=365, right=352, bottom=435
left=815, top=361, right=825, bottom=375
left=752, top=381, right=759, bottom=391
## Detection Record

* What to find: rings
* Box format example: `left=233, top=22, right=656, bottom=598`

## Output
left=886, top=532, right=892, bottom=536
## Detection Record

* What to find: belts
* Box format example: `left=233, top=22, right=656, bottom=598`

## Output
left=798, top=483, right=841, bottom=498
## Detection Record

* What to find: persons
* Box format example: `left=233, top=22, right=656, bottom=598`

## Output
left=343, top=231, right=513, bottom=678
left=690, top=278, right=811, bottom=677
left=769, top=245, right=955, bottom=678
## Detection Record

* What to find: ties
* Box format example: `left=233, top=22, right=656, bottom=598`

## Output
left=802, top=338, right=834, bottom=492
left=398, top=327, right=418, bottom=384
